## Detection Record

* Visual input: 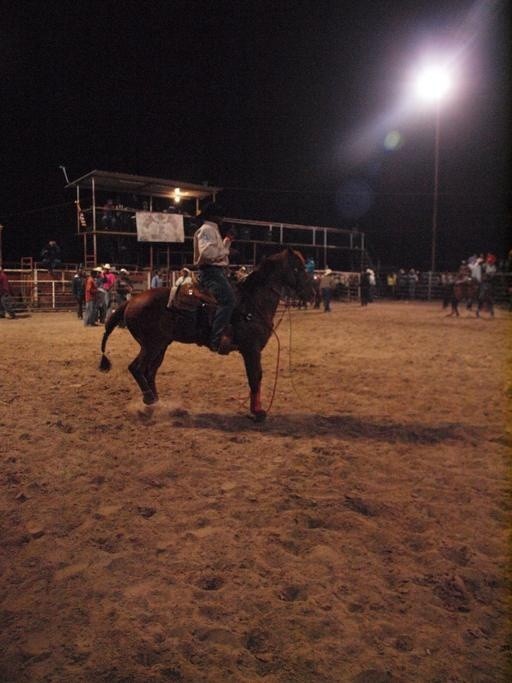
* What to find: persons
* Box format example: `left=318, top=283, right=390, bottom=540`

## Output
left=190, top=201, right=242, bottom=354
left=157, top=274, right=163, bottom=288
left=175, top=266, right=192, bottom=287
left=1, top=265, right=16, bottom=318
left=150, top=270, right=160, bottom=288
left=40, top=240, right=61, bottom=265
left=442, top=250, right=512, bottom=317
left=313, top=267, right=421, bottom=313
left=72, top=263, right=133, bottom=325
left=100, top=198, right=116, bottom=230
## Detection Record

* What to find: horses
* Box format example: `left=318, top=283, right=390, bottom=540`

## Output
left=98, top=243, right=318, bottom=424
left=439, top=283, right=495, bottom=317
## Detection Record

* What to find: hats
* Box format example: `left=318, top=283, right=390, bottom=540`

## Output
left=102, top=263, right=112, bottom=270
left=118, top=268, right=130, bottom=275
left=92, top=266, right=103, bottom=273
